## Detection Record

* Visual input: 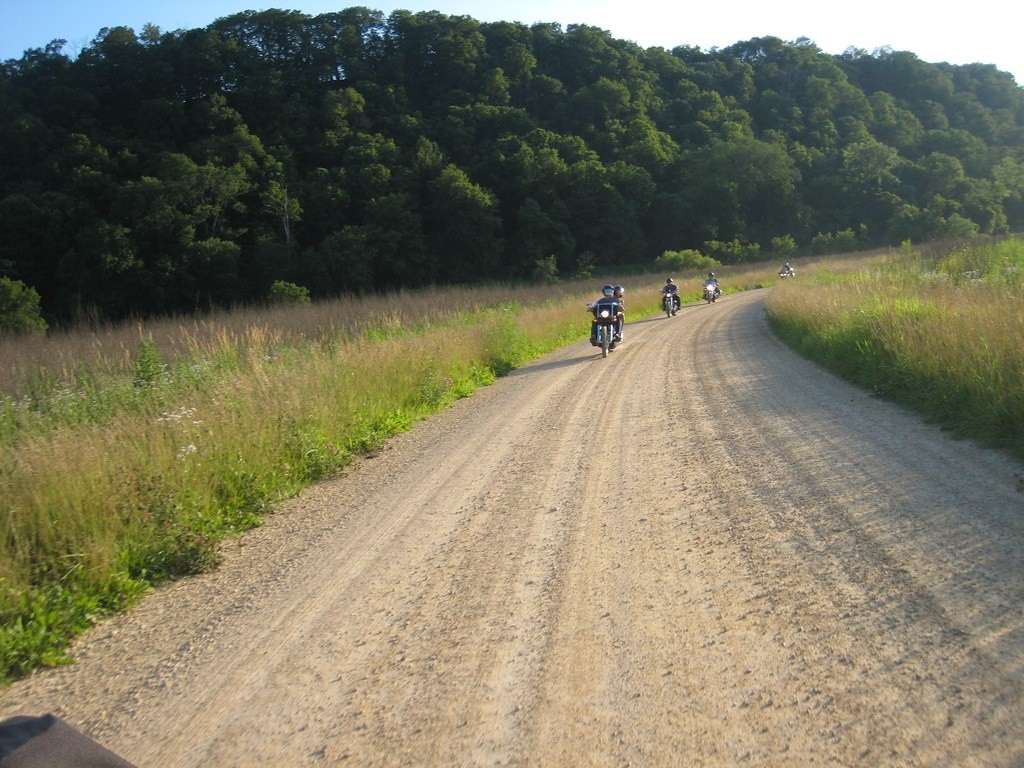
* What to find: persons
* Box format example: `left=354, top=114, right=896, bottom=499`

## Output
left=662, top=278, right=681, bottom=310
left=704, top=272, right=720, bottom=298
left=590, top=284, right=624, bottom=339
left=778, top=262, right=792, bottom=274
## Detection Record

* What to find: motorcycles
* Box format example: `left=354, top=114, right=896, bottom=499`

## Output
left=777, top=268, right=796, bottom=279
left=703, top=281, right=719, bottom=304
left=659, top=289, right=680, bottom=318
left=586, top=302, right=622, bottom=359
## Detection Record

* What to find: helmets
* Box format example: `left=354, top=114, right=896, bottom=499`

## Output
left=615, top=285, right=624, bottom=293
left=666, top=278, right=673, bottom=283
left=602, top=285, right=614, bottom=296
left=708, top=273, right=714, bottom=276
